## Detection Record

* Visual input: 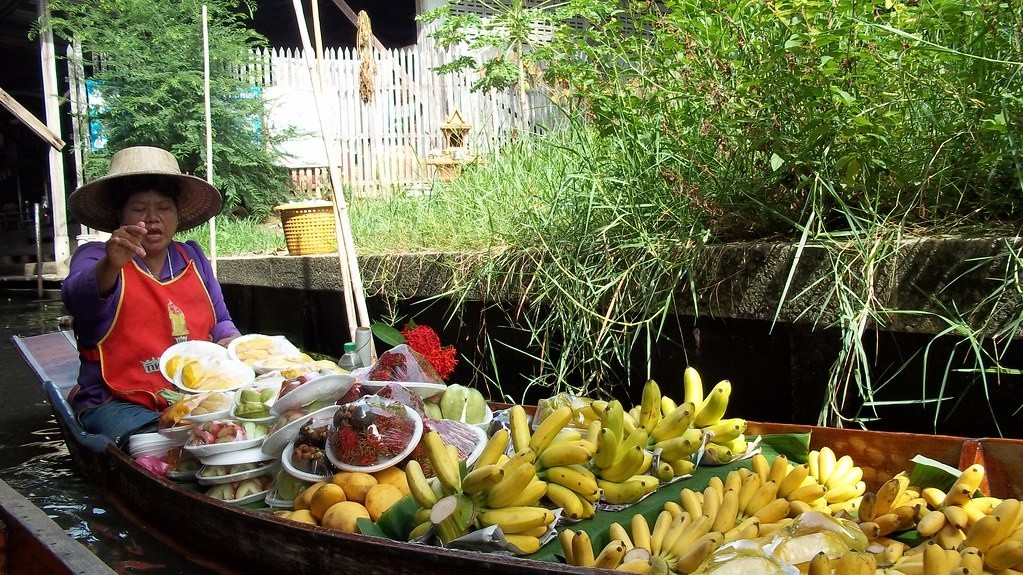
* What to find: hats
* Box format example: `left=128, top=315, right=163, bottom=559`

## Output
left=68, top=146, right=222, bottom=233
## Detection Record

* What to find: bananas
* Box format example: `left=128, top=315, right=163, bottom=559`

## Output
left=405, top=366, right=748, bottom=552
left=560, top=448, right=1023, bottom=575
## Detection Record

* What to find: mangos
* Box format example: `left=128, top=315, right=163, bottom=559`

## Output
left=270, top=465, right=411, bottom=534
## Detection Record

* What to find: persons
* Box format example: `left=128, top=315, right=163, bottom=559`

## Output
left=61, top=147, right=242, bottom=439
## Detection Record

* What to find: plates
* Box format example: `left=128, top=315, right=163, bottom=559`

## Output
left=129, top=334, right=488, bottom=509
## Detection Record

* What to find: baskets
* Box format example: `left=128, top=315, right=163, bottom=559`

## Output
left=273, top=200, right=350, bottom=255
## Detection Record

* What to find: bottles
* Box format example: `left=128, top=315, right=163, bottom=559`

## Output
left=338, top=343, right=362, bottom=370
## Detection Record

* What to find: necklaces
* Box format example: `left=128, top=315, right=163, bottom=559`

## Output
left=146, top=248, right=173, bottom=282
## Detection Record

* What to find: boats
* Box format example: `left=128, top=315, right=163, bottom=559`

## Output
left=13, top=330, right=1023, bottom=575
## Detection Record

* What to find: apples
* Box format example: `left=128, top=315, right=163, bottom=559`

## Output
left=161, top=374, right=334, bottom=502
